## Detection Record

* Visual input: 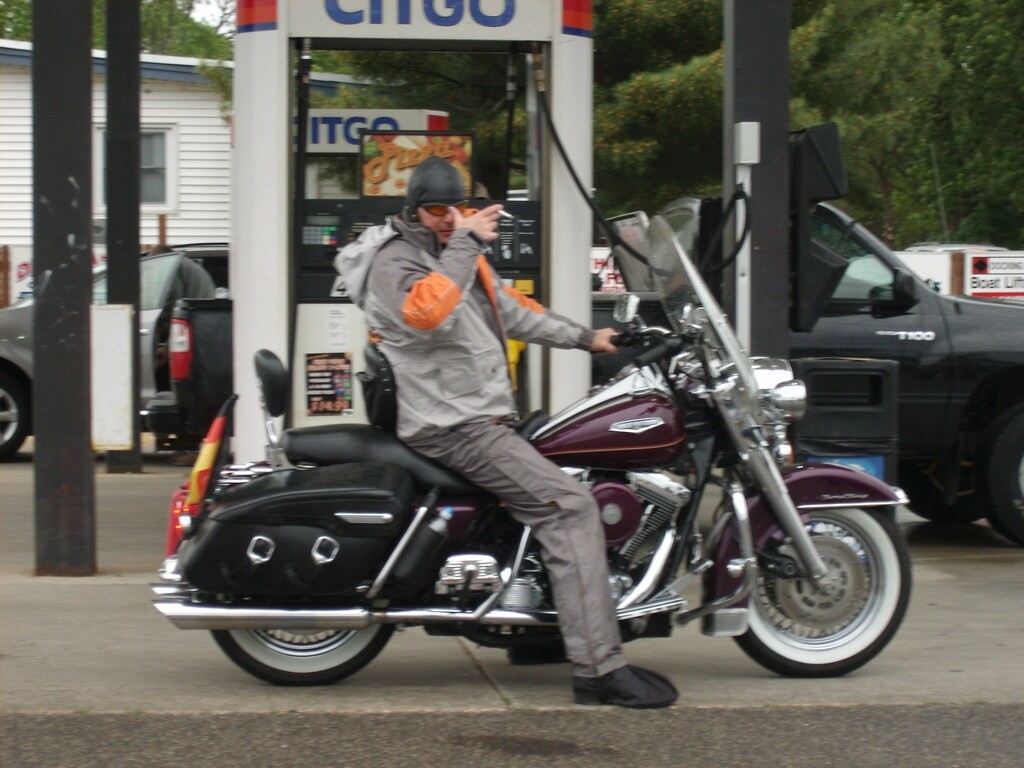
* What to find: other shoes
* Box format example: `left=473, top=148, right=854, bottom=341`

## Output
left=508, top=644, right=570, bottom=666
left=571, top=662, right=680, bottom=710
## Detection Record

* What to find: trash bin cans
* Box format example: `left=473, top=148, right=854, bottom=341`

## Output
left=785, top=357, right=899, bottom=520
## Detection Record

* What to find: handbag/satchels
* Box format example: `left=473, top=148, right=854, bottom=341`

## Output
left=354, top=342, right=398, bottom=435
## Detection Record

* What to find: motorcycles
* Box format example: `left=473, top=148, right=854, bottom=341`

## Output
left=142, top=214, right=914, bottom=688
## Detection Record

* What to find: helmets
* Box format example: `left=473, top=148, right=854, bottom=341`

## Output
left=407, top=155, right=466, bottom=213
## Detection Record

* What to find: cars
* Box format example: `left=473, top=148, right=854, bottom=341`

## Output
left=0, top=240, right=236, bottom=465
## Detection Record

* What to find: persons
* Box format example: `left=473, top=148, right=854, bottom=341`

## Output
left=147, top=244, right=217, bottom=451
left=332, top=157, right=679, bottom=710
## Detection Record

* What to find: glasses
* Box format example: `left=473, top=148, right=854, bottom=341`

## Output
left=420, top=201, right=466, bottom=217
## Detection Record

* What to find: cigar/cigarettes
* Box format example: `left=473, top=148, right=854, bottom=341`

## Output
left=496, top=210, right=514, bottom=220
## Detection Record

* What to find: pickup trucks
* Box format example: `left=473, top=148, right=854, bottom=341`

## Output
left=142, top=187, right=1024, bottom=556
